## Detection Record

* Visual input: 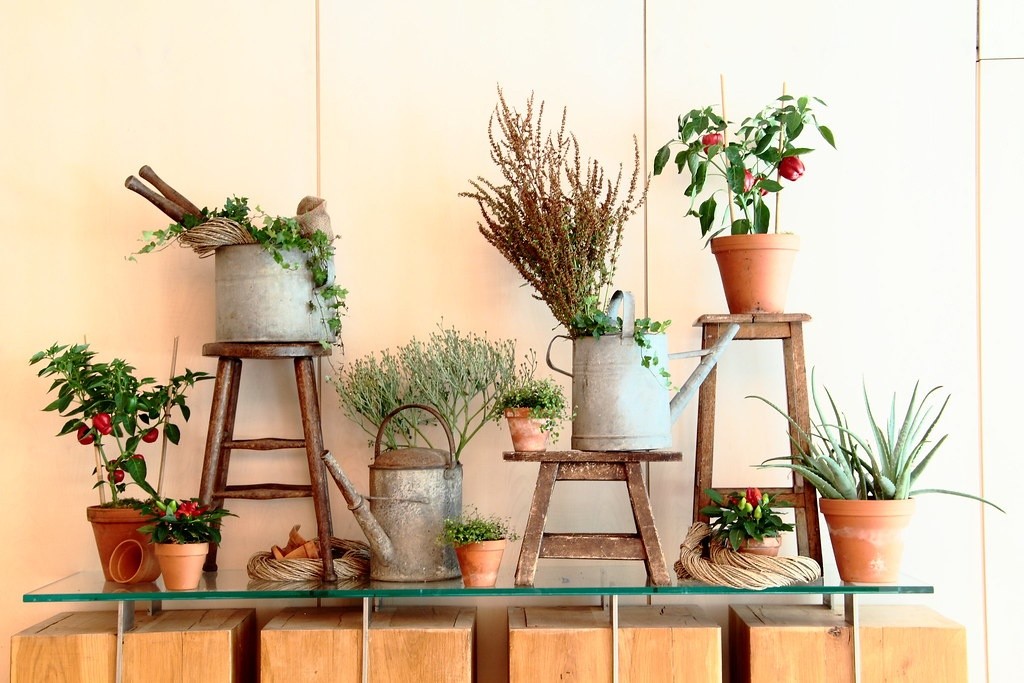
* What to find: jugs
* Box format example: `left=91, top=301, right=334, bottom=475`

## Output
left=320, top=405, right=466, bottom=584
left=547, top=291, right=741, bottom=453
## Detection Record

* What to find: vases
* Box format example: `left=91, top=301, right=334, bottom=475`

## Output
left=86, top=500, right=161, bottom=587
left=708, top=232, right=798, bottom=316
left=454, top=538, right=505, bottom=588
left=156, top=540, right=209, bottom=589
left=737, top=532, right=781, bottom=558
left=502, top=405, right=554, bottom=453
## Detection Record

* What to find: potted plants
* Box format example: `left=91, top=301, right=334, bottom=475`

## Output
left=435, top=506, right=523, bottom=553
left=747, top=364, right=1009, bottom=585
left=461, top=82, right=687, bottom=396
left=488, top=373, right=580, bottom=444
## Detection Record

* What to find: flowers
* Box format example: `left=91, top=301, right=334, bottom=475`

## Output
left=652, top=94, right=837, bottom=247
left=702, top=484, right=797, bottom=553
left=31, top=340, right=217, bottom=511
left=128, top=495, right=239, bottom=550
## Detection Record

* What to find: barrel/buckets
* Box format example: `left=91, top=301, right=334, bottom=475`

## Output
left=216, top=241, right=340, bottom=345
